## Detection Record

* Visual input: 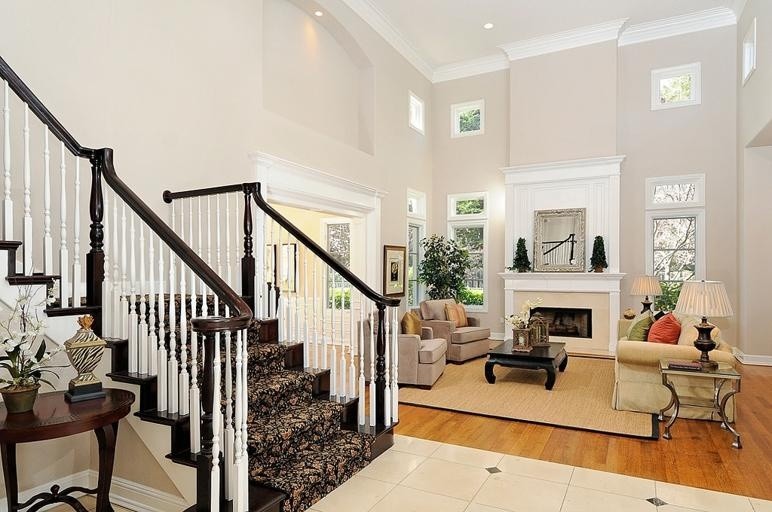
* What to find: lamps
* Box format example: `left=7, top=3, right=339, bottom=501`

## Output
left=674, top=278, right=735, bottom=369
left=629, top=274, right=663, bottom=314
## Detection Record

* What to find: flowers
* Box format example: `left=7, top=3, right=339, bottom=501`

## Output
left=498, top=295, right=545, bottom=328
left=0, top=260, right=70, bottom=390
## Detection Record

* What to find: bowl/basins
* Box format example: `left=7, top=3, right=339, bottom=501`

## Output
left=624, top=314, right=636, bottom=319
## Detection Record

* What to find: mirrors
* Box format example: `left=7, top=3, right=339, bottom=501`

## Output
left=532, top=209, right=587, bottom=272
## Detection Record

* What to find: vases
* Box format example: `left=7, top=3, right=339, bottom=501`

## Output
left=1, top=382, right=42, bottom=413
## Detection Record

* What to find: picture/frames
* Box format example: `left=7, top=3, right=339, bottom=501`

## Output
left=382, top=244, right=408, bottom=298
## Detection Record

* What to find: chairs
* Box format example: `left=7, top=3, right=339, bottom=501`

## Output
left=410, top=304, right=489, bottom=364
left=357, top=317, right=446, bottom=389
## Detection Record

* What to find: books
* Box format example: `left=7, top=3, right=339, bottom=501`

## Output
left=668, top=359, right=703, bottom=371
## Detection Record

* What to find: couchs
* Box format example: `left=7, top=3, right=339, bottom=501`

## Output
left=613, top=318, right=736, bottom=423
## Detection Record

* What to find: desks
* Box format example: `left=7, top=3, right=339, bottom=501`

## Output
left=655, top=358, right=743, bottom=450
left=0, top=386, right=137, bottom=511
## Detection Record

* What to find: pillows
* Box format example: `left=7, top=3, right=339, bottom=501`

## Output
left=402, top=312, right=423, bottom=341
left=419, top=296, right=456, bottom=323
left=625, top=309, right=682, bottom=345
left=367, top=308, right=402, bottom=335
left=444, top=303, right=469, bottom=327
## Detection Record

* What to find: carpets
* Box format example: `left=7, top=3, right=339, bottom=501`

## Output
left=399, top=340, right=662, bottom=442
